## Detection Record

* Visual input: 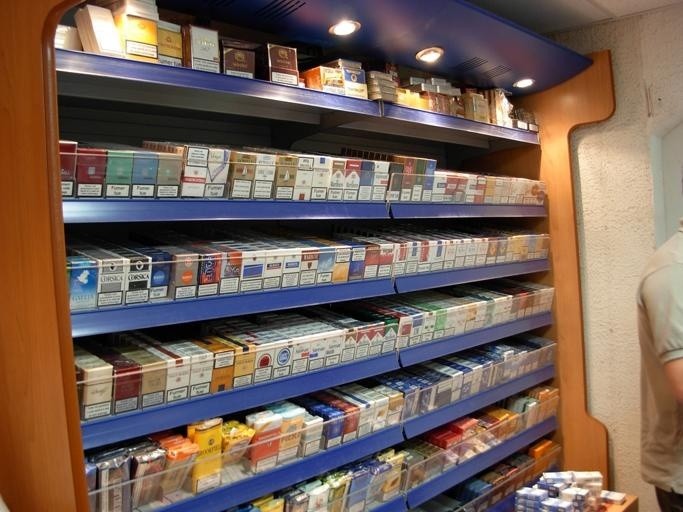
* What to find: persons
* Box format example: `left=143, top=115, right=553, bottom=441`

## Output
left=636, top=218, right=683, bottom=511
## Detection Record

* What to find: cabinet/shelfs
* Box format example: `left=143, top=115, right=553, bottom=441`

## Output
left=1, top=1, right=640, bottom=512
